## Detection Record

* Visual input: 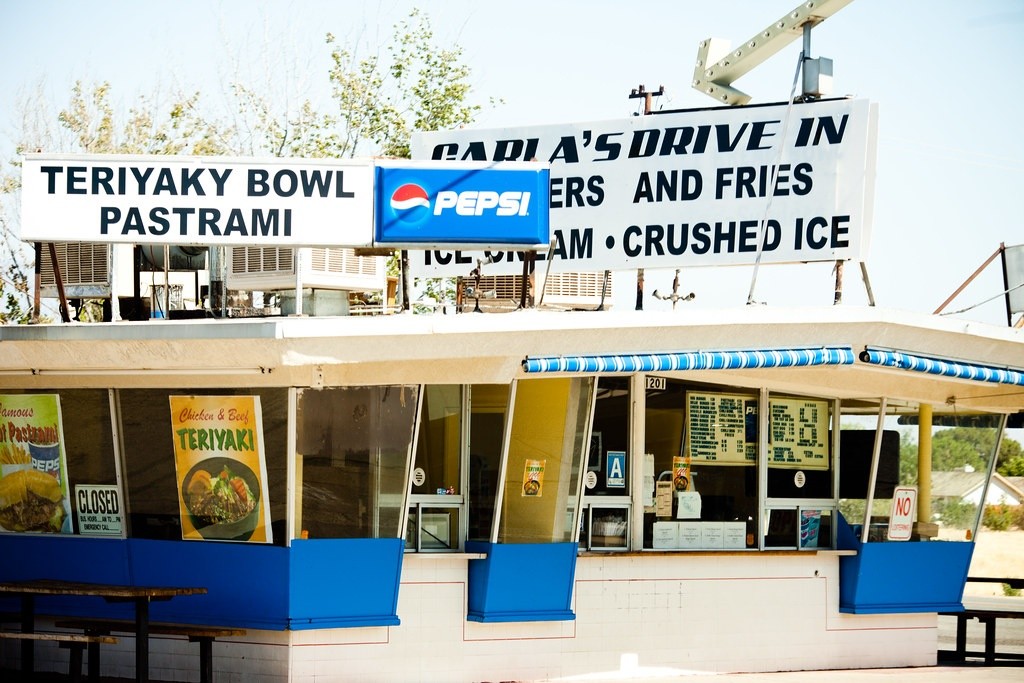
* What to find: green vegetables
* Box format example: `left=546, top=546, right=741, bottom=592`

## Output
left=214, top=464, right=255, bottom=503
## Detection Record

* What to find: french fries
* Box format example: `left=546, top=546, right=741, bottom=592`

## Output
left=0, top=442, right=32, bottom=466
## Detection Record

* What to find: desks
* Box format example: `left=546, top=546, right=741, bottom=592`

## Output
left=0, top=579, right=209, bottom=682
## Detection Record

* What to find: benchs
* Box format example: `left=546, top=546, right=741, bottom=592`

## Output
left=0, top=619, right=246, bottom=683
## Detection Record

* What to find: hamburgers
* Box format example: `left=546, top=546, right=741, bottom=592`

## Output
left=0, top=469, right=64, bottom=533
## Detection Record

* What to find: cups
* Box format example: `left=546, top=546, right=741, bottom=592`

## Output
left=27, top=440, right=61, bottom=485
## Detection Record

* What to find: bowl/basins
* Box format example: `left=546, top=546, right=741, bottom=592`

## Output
left=182, top=456, right=260, bottom=542
left=674, top=476, right=688, bottom=490
